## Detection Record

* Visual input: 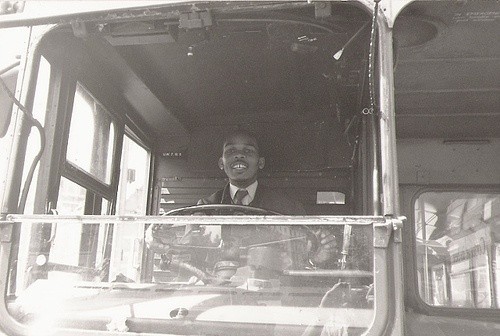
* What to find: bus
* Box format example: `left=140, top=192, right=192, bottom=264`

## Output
left=0, top=0, right=500, bottom=335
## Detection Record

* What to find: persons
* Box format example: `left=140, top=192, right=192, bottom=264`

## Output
left=144, top=126, right=339, bottom=280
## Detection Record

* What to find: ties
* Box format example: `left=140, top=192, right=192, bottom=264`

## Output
left=234, top=190, right=248, bottom=205
